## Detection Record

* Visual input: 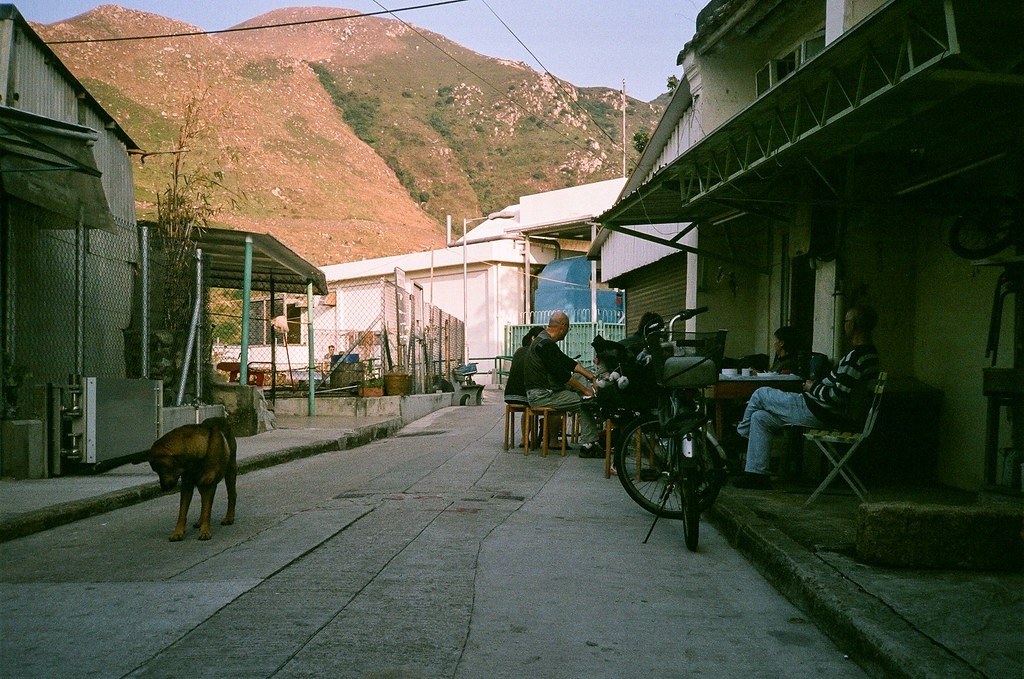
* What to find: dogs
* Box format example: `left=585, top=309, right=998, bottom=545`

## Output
left=130, top=416, right=238, bottom=541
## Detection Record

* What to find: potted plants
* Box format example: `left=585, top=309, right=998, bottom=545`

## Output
left=357, top=376, right=384, bottom=397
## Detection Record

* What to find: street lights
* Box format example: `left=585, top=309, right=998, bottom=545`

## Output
left=462, top=210, right=515, bottom=386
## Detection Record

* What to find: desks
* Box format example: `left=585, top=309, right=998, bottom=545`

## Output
left=706, top=379, right=802, bottom=446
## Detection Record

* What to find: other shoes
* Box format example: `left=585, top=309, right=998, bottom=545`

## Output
left=740, top=473, right=772, bottom=490
left=724, top=434, right=748, bottom=448
left=579, top=441, right=606, bottom=458
left=519, top=441, right=530, bottom=448
left=609, top=462, right=618, bottom=475
left=549, top=444, right=572, bottom=450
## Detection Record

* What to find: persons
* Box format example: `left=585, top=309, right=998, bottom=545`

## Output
left=503, top=312, right=607, bottom=458
left=717, top=305, right=879, bottom=490
left=769, top=325, right=797, bottom=374
left=597, top=314, right=665, bottom=413
left=324, top=345, right=335, bottom=359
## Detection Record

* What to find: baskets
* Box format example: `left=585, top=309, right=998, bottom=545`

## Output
left=597, top=366, right=642, bottom=425
left=647, top=329, right=728, bottom=387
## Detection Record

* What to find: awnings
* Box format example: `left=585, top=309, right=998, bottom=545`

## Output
left=150, top=221, right=326, bottom=415
left=0, top=104, right=119, bottom=236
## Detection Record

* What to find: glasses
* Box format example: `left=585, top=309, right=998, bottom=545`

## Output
left=331, top=349, right=334, bottom=350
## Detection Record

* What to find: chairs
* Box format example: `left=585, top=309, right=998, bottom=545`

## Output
left=802, top=370, right=889, bottom=506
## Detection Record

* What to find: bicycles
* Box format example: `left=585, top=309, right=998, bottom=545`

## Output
left=613, top=306, right=730, bottom=550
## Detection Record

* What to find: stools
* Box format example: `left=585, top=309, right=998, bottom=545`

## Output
left=528, top=407, right=567, bottom=458
left=505, top=402, right=536, bottom=451
left=605, top=415, right=658, bottom=482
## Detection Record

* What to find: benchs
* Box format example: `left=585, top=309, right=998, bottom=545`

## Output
left=449, top=370, right=485, bottom=407
